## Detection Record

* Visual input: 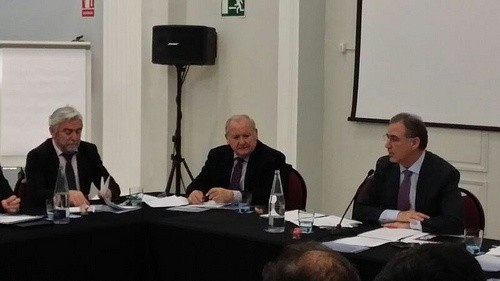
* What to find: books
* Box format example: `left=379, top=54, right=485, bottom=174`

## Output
left=84, top=175, right=141, bottom=214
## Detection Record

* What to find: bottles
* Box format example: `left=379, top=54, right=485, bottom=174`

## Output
left=53, top=164, right=70, bottom=224
left=268, top=170, right=286, bottom=233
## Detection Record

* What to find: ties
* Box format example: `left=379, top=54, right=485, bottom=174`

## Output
left=61, top=152, right=77, bottom=191
left=231, top=156, right=244, bottom=191
left=396, top=169, right=413, bottom=211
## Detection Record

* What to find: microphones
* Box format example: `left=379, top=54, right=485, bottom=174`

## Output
left=326, top=169, right=375, bottom=236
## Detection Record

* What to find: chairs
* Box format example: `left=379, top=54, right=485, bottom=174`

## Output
left=285, top=167, right=309, bottom=210
left=459, top=187, right=484, bottom=238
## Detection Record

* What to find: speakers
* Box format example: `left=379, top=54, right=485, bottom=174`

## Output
left=150, top=25, right=217, bottom=66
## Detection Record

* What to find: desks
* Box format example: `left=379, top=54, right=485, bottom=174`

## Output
left=0, top=192, right=500, bottom=281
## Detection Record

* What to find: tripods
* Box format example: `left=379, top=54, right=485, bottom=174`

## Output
left=165, top=66, right=195, bottom=195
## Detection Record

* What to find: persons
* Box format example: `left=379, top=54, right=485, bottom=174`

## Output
left=0, top=164, right=21, bottom=215
left=262, top=241, right=361, bottom=281
left=23, top=106, right=120, bottom=213
left=186, top=115, right=290, bottom=207
left=372, top=241, right=487, bottom=281
left=352, top=112, right=465, bottom=235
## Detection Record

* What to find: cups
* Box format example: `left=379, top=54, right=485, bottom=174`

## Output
left=464, top=228, right=483, bottom=255
left=238, top=192, right=252, bottom=214
left=298, top=209, right=315, bottom=234
left=46, top=199, right=54, bottom=220
left=129, top=186, right=144, bottom=207
